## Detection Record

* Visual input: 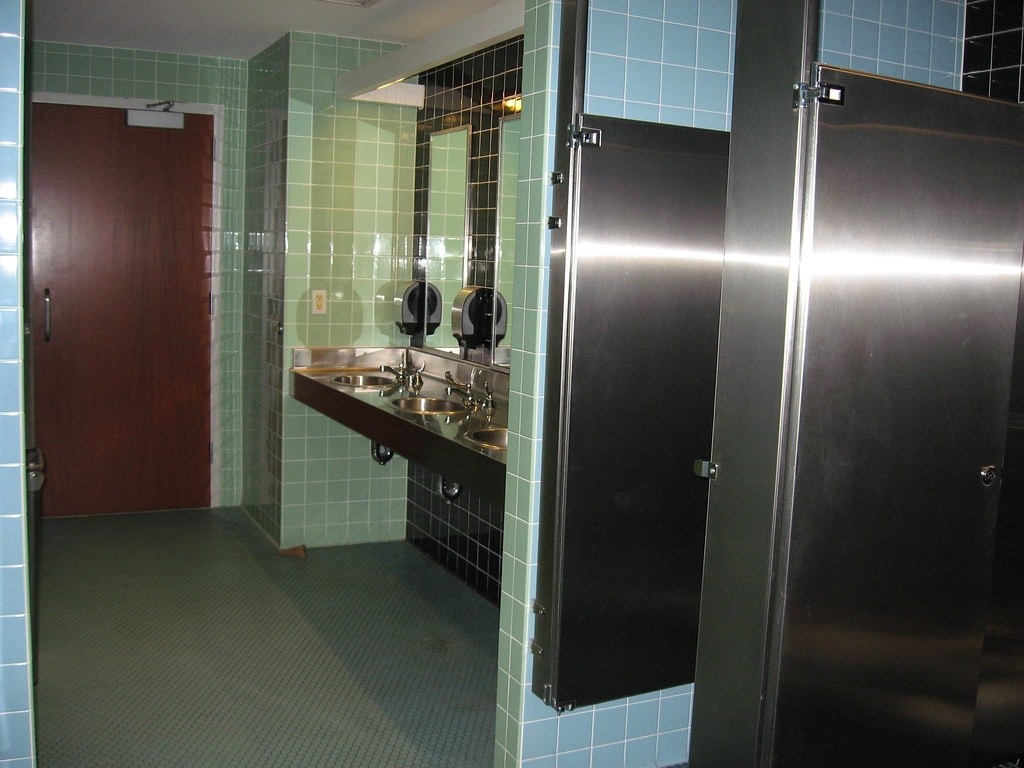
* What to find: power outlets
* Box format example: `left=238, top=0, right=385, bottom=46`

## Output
left=312, top=289, right=327, bottom=314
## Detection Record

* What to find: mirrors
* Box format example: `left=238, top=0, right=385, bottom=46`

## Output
left=425, top=123, right=472, bottom=358
left=491, top=113, right=520, bottom=376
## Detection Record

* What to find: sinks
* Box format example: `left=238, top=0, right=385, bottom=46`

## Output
left=463, top=428, right=507, bottom=450
left=387, top=396, right=469, bottom=414
left=328, top=374, right=399, bottom=390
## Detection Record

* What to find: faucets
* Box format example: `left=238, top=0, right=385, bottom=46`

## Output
left=379, top=382, right=424, bottom=397
left=443, top=384, right=481, bottom=410
left=379, top=363, right=406, bottom=382
left=443, top=404, right=495, bottom=429
